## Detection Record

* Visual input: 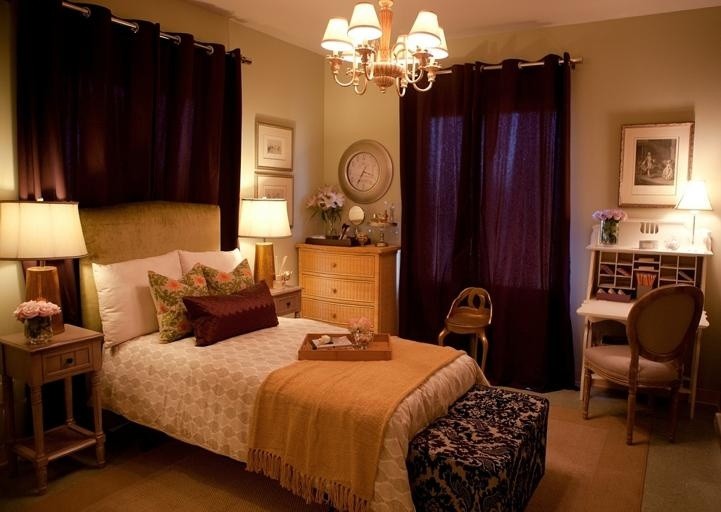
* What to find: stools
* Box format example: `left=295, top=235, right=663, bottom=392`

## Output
left=448, top=379, right=551, bottom=504
left=405, top=413, right=533, bottom=510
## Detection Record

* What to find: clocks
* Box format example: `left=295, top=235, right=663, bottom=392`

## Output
left=335, top=139, right=395, bottom=204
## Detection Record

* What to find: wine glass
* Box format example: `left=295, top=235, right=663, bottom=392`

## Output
left=354, top=331, right=373, bottom=351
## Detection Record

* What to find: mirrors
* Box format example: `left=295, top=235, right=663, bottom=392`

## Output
left=347, top=204, right=367, bottom=241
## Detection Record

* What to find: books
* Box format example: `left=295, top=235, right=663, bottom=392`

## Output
left=310, top=335, right=356, bottom=351
left=597, top=264, right=694, bottom=296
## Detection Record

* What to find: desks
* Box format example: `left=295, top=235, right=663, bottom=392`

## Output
left=576, top=221, right=716, bottom=423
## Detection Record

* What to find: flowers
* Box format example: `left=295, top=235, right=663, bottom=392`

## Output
left=590, top=207, right=628, bottom=245
left=12, top=297, right=63, bottom=347
left=304, top=184, right=349, bottom=236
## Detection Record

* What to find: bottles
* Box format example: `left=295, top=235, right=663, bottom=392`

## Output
left=373, top=202, right=395, bottom=222
left=271, top=274, right=285, bottom=290
left=702, top=230, right=713, bottom=253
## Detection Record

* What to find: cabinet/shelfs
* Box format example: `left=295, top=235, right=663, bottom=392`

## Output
left=294, top=241, right=401, bottom=338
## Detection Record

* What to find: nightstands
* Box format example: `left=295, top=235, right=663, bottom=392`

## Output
left=1, top=324, right=107, bottom=497
left=268, top=285, right=305, bottom=319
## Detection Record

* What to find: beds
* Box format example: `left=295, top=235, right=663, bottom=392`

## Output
left=74, top=201, right=495, bottom=512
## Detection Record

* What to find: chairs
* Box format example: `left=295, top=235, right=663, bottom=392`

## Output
left=580, top=283, right=706, bottom=445
left=436, top=286, right=495, bottom=375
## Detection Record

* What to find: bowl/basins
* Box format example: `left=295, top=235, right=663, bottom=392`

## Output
left=663, top=240, right=682, bottom=251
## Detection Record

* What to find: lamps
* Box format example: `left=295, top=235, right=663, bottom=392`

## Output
left=0, top=199, right=92, bottom=338
left=238, top=198, right=295, bottom=288
left=320, top=1, right=452, bottom=99
left=674, top=180, right=714, bottom=252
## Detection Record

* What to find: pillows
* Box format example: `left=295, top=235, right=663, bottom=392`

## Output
left=201, top=256, right=256, bottom=299
left=181, top=279, right=281, bottom=346
left=144, top=262, right=210, bottom=345
left=178, top=246, right=247, bottom=280
left=92, top=248, right=184, bottom=350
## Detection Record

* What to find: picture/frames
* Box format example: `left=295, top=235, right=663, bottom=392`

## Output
left=617, top=121, right=696, bottom=208
left=252, top=170, right=298, bottom=230
left=256, top=122, right=294, bottom=172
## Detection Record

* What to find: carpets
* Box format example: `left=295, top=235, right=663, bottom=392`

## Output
left=88, top=402, right=652, bottom=510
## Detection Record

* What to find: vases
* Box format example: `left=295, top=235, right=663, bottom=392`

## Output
left=600, top=219, right=621, bottom=248
left=319, top=210, right=344, bottom=240
left=22, top=316, right=54, bottom=347
